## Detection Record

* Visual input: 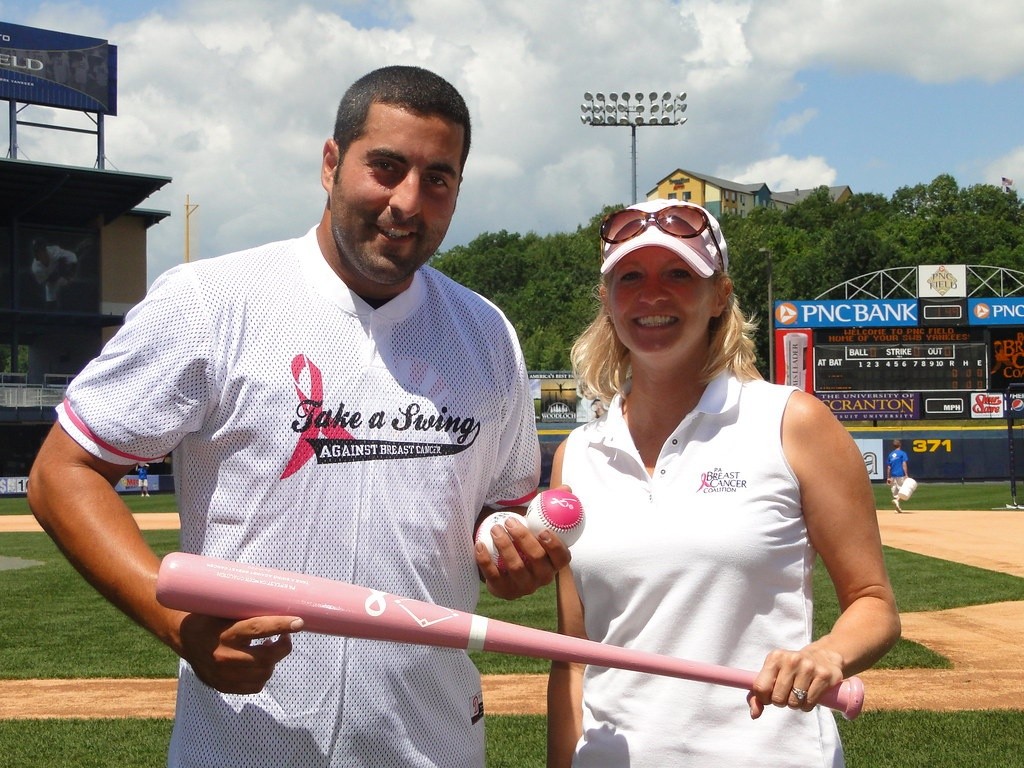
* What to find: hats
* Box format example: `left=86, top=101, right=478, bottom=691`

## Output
left=598, top=197, right=729, bottom=279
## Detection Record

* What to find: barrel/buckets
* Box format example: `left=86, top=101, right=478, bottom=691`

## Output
left=899, top=477, right=917, bottom=502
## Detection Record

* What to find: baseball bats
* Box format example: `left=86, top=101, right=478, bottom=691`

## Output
left=153, top=551, right=866, bottom=721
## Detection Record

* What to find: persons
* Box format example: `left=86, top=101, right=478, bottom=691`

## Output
left=545, top=198, right=903, bottom=768
left=31, top=237, right=80, bottom=311
left=24, top=65, right=574, bottom=768
left=135, top=462, right=151, bottom=498
left=886, top=440, right=908, bottom=513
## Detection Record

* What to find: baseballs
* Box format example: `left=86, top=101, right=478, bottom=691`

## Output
left=525, top=489, right=588, bottom=550
left=474, top=509, right=529, bottom=567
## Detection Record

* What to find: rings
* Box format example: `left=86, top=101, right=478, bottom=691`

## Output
left=791, top=687, right=807, bottom=700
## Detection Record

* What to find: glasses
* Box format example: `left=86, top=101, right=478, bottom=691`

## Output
left=600, top=205, right=725, bottom=275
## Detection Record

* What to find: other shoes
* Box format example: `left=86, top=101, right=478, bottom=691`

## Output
left=145, top=494, right=150, bottom=497
left=141, top=494, right=145, bottom=497
left=892, top=499, right=901, bottom=514
